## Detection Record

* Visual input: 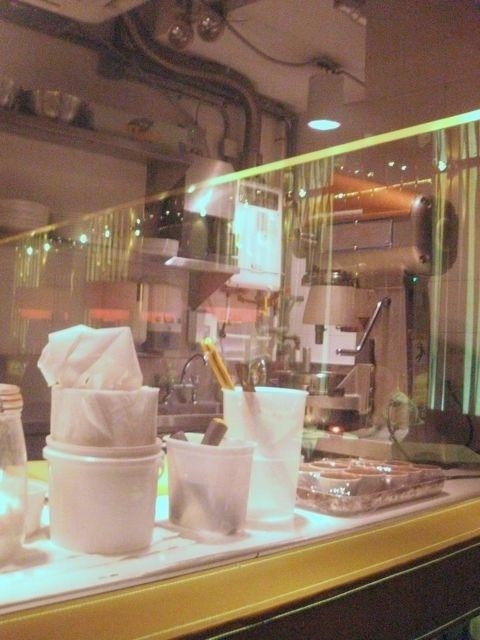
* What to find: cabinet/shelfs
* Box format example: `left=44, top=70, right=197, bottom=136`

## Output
left=0, top=104, right=245, bottom=282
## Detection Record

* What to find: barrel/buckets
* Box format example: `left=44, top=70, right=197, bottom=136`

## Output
left=218, top=385, right=308, bottom=527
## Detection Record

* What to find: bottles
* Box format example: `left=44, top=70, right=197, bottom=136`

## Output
left=0, top=383, right=27, bottom=562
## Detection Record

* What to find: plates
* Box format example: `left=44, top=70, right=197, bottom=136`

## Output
left=0, top=197, right=98, bottom=243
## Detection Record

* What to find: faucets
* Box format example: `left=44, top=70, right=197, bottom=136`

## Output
left=180, top=355, right=208, bottom=382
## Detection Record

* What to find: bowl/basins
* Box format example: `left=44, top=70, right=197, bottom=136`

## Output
left=21, top=89, right=84, bottom=125
left=298, top=459, right=442, bottom=497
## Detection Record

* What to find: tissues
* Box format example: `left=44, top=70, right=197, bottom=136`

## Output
left=37, top=324, right=159, bottom=448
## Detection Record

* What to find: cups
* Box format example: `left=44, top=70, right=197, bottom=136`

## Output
left=162, top=386, right=309, bottom=544
